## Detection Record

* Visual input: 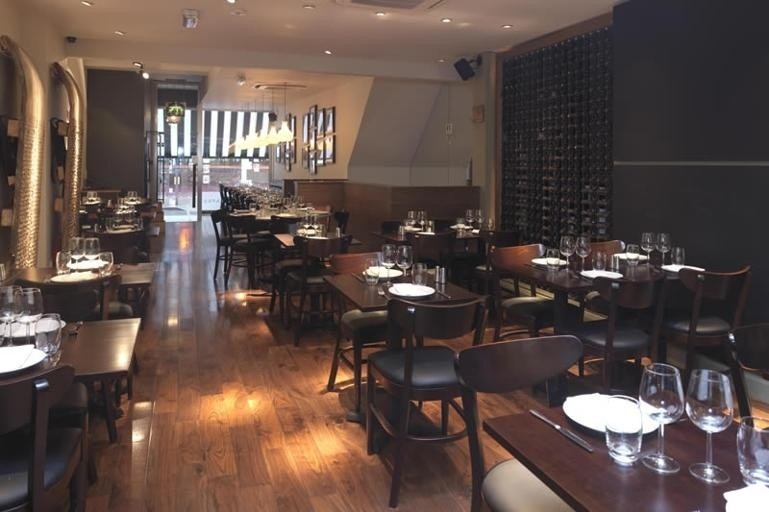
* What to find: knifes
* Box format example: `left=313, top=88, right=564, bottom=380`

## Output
left=529, top=407, right=592, bottom=455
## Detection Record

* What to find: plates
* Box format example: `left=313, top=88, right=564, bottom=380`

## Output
left=48, top=259, right=109, bottom=285
left=615, top=251, right=705, bottom=275
left=388, top=284, right=436, bottom=299
left=529, top=257, right=629, bottom=280
left=564, top=392, right=660, bottom=435
left=0, top=317, right=66, bottom=378
left=361, top=268, right=403, bottom=279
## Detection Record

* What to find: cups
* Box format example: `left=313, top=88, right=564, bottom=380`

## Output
left=411, top=263, right=428, bottom=286
left=734, top=413, right=768, bottom=488
left=593, top=251, right=606, bottom=270
left=55, top=250, right=114, bottom=274
left=627, top=243, right=640, bottom=266
left=397, top=218, right=495, bottom=236
left=670, top=247, right=685, bottom=265
left=603, top=394, right=643, bottom=467
left=33, top=314, right=63, bottom=356
left=547, top=249, right=559, bottom=271
left=434, top=264, right=449, bottom=285
left=364, top=257, right=381, bottom=286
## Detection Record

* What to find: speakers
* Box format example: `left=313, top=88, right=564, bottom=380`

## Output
left=455, top=58, right=475, bottom=81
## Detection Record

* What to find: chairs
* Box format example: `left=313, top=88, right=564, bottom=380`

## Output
left=255, top=214, right=301, bottom=284
left=656, top=262, right=761, bottom=425
left=487, top=239, right=584, bottom=340
left=209, top=171, right=351, bottom=232
left=276, top=234, right=352, bottom=345
left=2, top=361, right=86, bottom=510
left=212, top=213, right=255, bottom=278
left=223, top=215, right=273, bottom=289
left=325, top=249, right=424, bottom=423
left=577, top=272, right=667, bottom=395
left=570, top=237, right=630, bottom=266
left=455, top=331, right=588, bottom=511
left=77, top=189, right=166, bottom=331
left=360, top=294, right=488, bottom=510
left=12, top=275, right=133, bottom=445
left=371, top=215, right=514, bottom=290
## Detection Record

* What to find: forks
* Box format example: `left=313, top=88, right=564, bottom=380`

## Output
left=377, top=287, right=391, bottom=302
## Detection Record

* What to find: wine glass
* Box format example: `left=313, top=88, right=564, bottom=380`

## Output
left=244, top=192, right=320, bottom=237
left=380, top=242, right=396, bottom=285
left=687, top=366, right=730, bottom=486
left=576, top=236, right=591, bottom=273
left=68, top=237, right=83, bottom=273
left=85, top=237, right=99, bottom=272
left=396, top=244, right=413, bottom=283
left=641, top=231, right=656, bottom=267
left=560, top=235, right=576, bottom=274
left=407, top=208, right=486, bottom=228
left=85, top=190, right=139, bottom=230
left=656, top=232, right=671, bottom=267
left=0, top=285, right=23, bottom=347
left=640, top=359, right=686, bottom=480
left=14, top=288, right=44, bottom=346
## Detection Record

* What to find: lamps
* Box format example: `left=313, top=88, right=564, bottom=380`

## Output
left=452, top=53, right=483, bottom=82
left=236, top=82, right=296, bottom=150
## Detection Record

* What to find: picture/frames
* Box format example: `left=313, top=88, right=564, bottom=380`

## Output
left=301, top=103, right=338, bottom=177
left=275, top=113, right=297, bottom=175
